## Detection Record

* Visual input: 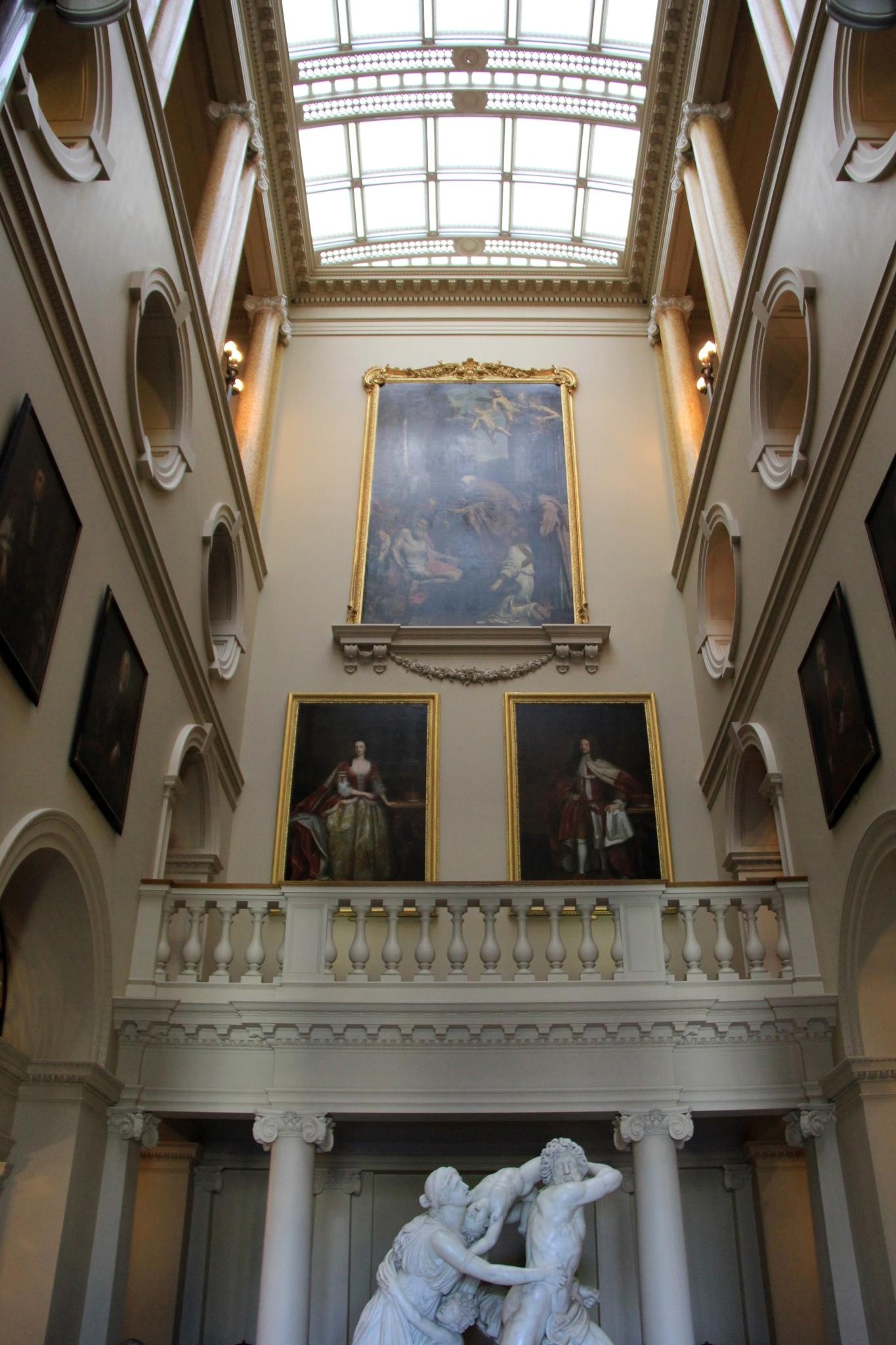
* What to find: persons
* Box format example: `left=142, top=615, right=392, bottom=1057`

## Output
left=352, top=1137, right=622, bottom=1345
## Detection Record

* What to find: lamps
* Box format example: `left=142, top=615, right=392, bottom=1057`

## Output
left=224, top=341, right=243, bottom=399
left=697, top=341, right=717, bottom=406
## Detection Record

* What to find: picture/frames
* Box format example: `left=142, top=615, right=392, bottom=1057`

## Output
left=347, top=356, right=588, bottom=628
left=68, top=583, right=149, bottom=836
left=269, top=690, right=441, bottom=917
left=503, top=690, right=679, bottom=917
left=797, top=580, right=882, bottom=830
left=0, top=394, right=85, bottom=707
left=863, top=455, right=896, bottom=640
